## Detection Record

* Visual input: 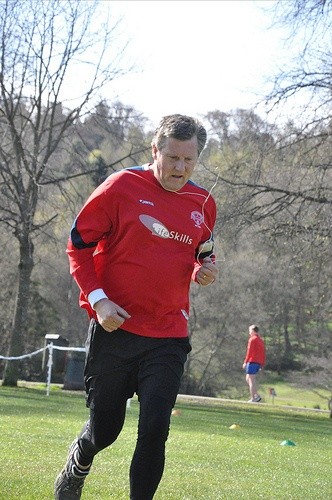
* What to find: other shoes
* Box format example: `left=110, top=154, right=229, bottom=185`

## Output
left=248, top=395, right=261, bottom=403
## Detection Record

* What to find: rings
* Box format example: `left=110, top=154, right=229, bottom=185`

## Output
left=203, top=275, right=207, bottom=279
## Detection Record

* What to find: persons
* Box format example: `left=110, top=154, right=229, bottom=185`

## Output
left=53, top=114, right=218, bottom=500
left=242, top=325, right=266, bottom=402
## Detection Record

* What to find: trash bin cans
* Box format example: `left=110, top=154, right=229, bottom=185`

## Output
left=63, top=346, right=85, bottom=390
left=41, top=333, right=70, bottom=374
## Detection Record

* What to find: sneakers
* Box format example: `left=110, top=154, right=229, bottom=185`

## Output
left=54, top=437, right=94, bottom=500
